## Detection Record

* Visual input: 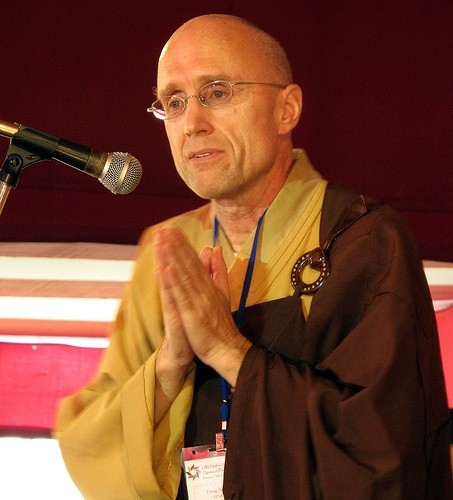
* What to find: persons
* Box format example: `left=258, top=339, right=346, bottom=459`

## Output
left=55, top=14, right=453, bottom=500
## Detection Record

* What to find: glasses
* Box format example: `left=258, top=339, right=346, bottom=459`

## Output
left=146, top=80, right=286, bottom=122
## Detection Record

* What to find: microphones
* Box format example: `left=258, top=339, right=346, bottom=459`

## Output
left=1, top=120, right=141, bottom=194
